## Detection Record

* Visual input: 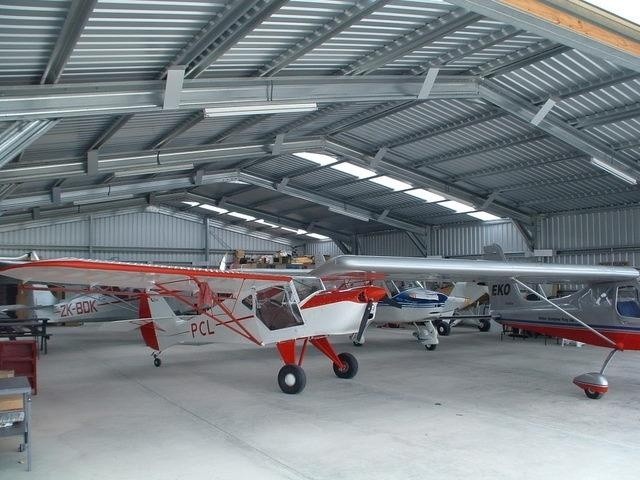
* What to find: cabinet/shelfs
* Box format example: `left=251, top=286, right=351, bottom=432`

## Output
left=0, top=338, right=37, bottom=395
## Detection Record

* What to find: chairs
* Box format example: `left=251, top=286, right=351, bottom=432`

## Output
left=618, top=300, right=640, bottom=319
left=258, top=298, right=304, bottom=328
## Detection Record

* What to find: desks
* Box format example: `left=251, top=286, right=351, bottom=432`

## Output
left=0, top=376, right=31, bottom=472
